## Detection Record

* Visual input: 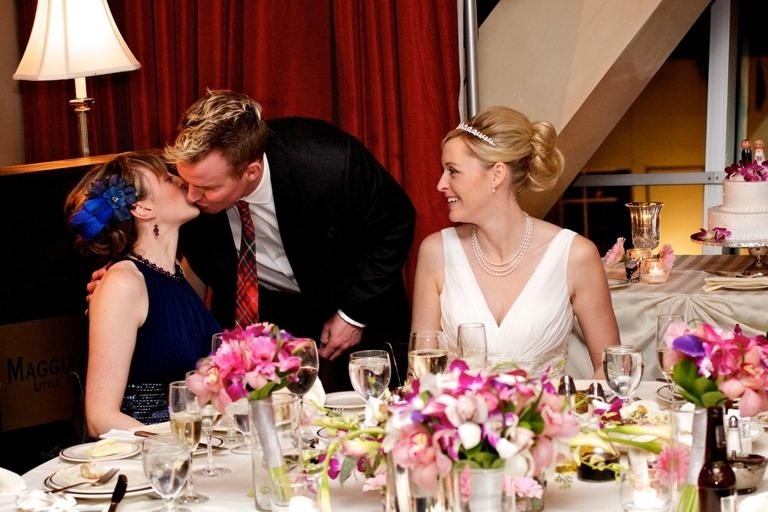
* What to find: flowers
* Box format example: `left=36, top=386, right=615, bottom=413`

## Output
left=658, top=321, right=768, bottom=512
left=301, top=360, right=664, bottom=512
left=657, top=243, right=676, bottom=272
left=605, top=237, right=629, bottom=265
left=186, top=322, right=311, bottom=501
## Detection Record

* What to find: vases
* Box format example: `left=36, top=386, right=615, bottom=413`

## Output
left=249, top=392, right=302, bottom=512
left=385, top=467, right=545, bottom=512
left=668, top=399, right=724, bottom=512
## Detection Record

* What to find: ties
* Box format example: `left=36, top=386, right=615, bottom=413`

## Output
left=233, top=199, right=260, bottom=326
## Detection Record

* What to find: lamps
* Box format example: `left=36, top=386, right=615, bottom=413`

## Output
left=11, top=0, right=142, bottom=156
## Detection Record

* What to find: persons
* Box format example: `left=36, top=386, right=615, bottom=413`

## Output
left=410, top=107, right=624, bottom=378
left=64, top=151, right=208, bottom=439
left=82, top=86, right=417, bottom=391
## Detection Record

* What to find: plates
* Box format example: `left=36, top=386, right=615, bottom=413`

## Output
left=43, top=460, right=154, bottom=499
left=324, top=387, right=368, bottom=411
left=607, top=277, right=630, bottom=288
left=59, top=439, right=143, bottom=461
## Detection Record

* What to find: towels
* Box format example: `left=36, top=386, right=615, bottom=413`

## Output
left=702, top=272, right=768, bottom=292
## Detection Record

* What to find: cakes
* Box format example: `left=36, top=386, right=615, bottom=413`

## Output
left=707, top=137, right=768, bottom=240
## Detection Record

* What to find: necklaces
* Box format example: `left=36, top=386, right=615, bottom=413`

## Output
left=474, top=211, right=528, bottom=265
left=470, top=221, right=534, bottom=278
left=128, top=249, right=185, bottom=280
left=472, top=217, right=530, bottom=271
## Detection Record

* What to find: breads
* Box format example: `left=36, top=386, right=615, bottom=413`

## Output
left=80, top=462, right=100, bottom=479
left=84, top=438, right=118, bottom=456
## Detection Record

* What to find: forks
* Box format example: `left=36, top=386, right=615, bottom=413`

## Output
left=45, top=468, right=118, bottom=496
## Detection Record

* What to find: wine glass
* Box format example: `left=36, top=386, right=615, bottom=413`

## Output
left=143, top=375, right=768, bottom=510
left=453, top=322, right=490, bottom=375
left=345, top=349, right=398, bottom=404
left=281, top=338, right=319, bottom=437
left=600, top=341, right=645, bottom=400
left=405, top=330, right=447, bottom=378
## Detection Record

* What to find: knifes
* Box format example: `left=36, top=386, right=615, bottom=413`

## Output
left=107, top=474, right=127, bottom=512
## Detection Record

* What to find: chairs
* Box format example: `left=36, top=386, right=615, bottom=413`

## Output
left=60, top=360, right=196, bottom=442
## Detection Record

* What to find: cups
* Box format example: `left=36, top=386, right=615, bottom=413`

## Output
left=642, top=257, right=671, bottom=284
left=624, top=249, right=641, bottom=282
left=626, top=201, right=664, bottom=250
